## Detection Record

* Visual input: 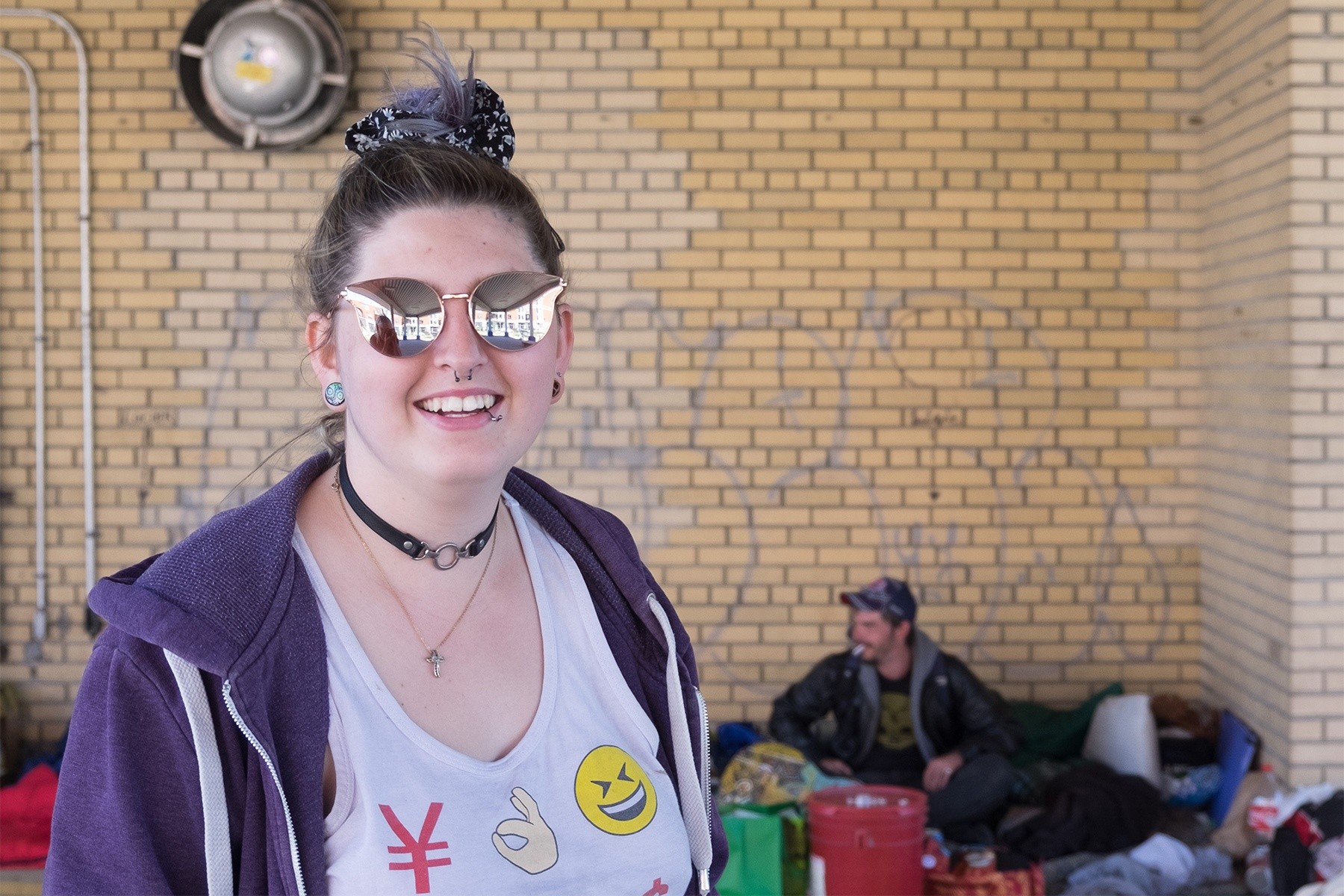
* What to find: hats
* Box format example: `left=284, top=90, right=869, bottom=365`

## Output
left=839, top=577, right=917, bottom=624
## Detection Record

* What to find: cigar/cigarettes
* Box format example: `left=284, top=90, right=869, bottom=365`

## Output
left=851, top=643, right=866, bottom=658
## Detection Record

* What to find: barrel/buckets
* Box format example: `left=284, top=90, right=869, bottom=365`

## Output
left=807, top=785, right=928, bottom=896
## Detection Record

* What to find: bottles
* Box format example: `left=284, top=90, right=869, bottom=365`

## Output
left=1248, top=765, right=1276, bottom=844
left=1248, top=844, right=1272, bottom=892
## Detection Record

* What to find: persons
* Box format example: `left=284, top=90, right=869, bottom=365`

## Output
left=768, top=577, right=1026, bottom=829
left=40, top=80, right=728, bottom=896
left=369, top=315, right=401, bottom=357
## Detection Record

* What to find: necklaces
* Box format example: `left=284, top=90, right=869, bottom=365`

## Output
left=331, top=452, right=500, bottom=678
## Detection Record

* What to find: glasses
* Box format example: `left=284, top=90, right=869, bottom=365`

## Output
left=326, top=271, right=568, bottom=358
